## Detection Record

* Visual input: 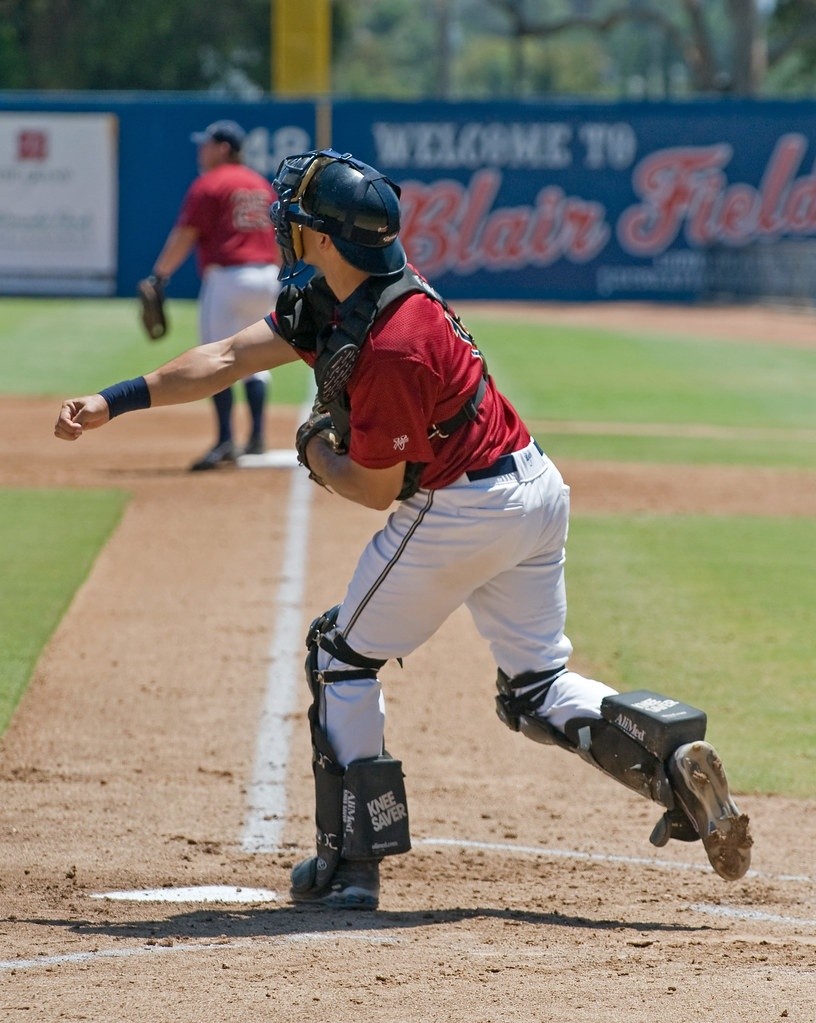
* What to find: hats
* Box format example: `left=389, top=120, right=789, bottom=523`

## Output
left=187, top=119, right=245, bottom=149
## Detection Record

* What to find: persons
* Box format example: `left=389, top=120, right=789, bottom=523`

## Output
left=54, top=149, right=754, bottom=909
left=137, top=120, right=278, bottom=474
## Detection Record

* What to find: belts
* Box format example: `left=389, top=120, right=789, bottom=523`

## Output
left=466, top=436, right=544, bottom=486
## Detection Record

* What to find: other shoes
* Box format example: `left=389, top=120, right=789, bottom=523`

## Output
left=190, top=442, right=234, bottom=471
left=244, top=441, right=263, bottom=455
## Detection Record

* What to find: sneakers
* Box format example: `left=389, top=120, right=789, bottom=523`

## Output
left=666, top=740, right=752, bottom=881
left=289, top=857, right=378, bottom=912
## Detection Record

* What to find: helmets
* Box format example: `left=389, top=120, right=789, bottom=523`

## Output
left=272, top=151, right=409, bottom=280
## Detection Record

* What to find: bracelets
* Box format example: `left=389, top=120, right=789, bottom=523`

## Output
left=97, top=375, right=152, bottom=421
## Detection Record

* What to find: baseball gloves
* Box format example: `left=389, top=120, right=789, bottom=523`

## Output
left=293, top=392, right=349, bottom=487
left=136, top=274, right=167, bottom=340
